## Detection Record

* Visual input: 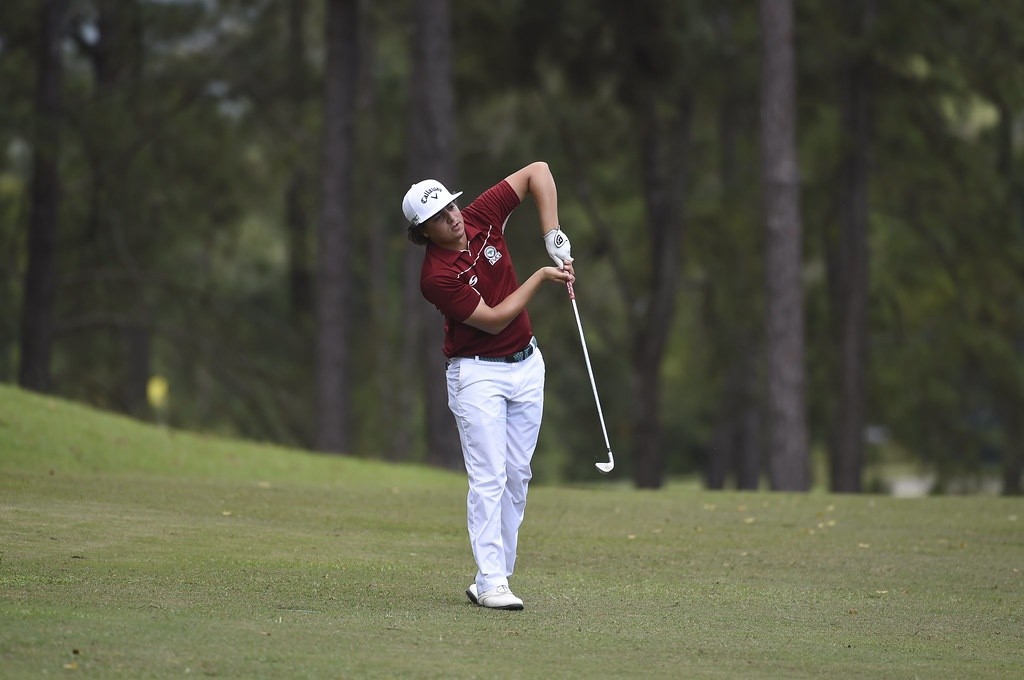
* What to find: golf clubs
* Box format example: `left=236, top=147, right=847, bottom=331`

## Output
left=564, top=268, right=615, bottom=474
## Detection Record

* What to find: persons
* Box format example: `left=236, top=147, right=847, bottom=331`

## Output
left=400, top=162, right=573, bottom=609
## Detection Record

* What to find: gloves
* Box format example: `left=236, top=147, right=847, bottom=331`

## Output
left=542, top=225, right=575, bottom=270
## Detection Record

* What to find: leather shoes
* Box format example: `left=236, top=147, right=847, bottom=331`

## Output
left=477, top=585, right=524, bottom=610
left=466, top=583, right=479, bottom=605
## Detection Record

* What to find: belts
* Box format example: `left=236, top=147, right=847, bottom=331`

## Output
left=460, top=344, right=533, bottom=363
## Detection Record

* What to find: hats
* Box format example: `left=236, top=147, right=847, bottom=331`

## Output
left=402, top=179, right=464, bottom=226
